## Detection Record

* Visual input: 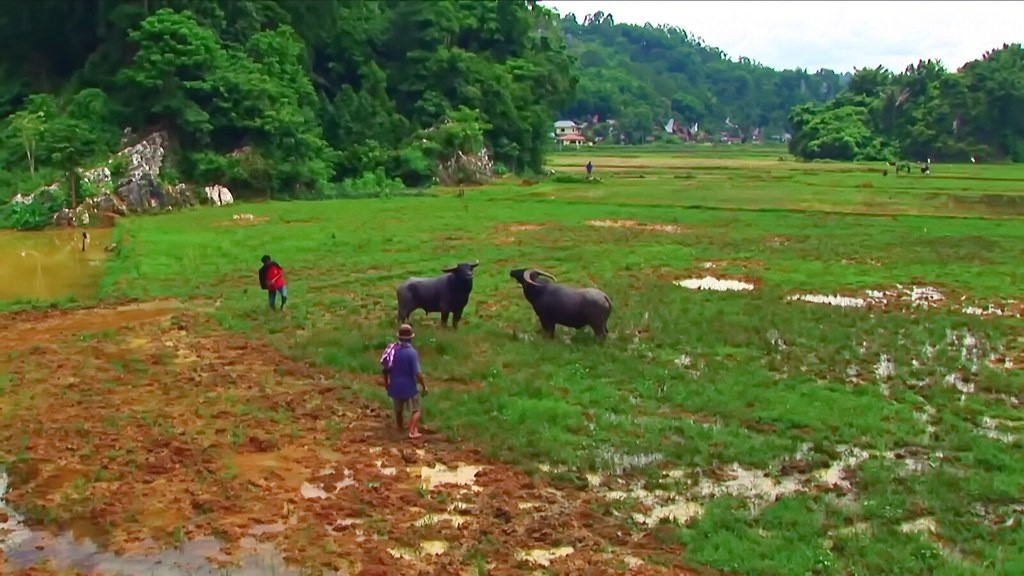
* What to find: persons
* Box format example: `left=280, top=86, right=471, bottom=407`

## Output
left=382, top=323, right=430, bottom=438
left=259, top=254, right=288, bottom=309
left=586, top=161, right=592, bottom=179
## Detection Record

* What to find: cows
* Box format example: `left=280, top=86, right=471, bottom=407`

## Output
left=396, top=260, right=479, bottom=331
left=510, top=267, right=612, bottom=343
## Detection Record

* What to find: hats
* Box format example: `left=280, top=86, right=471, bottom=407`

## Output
left=396, top=324, right=415, bottom=339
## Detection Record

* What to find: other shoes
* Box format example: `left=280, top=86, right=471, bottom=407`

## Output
left=409, top=432, right=422, bottom=438
